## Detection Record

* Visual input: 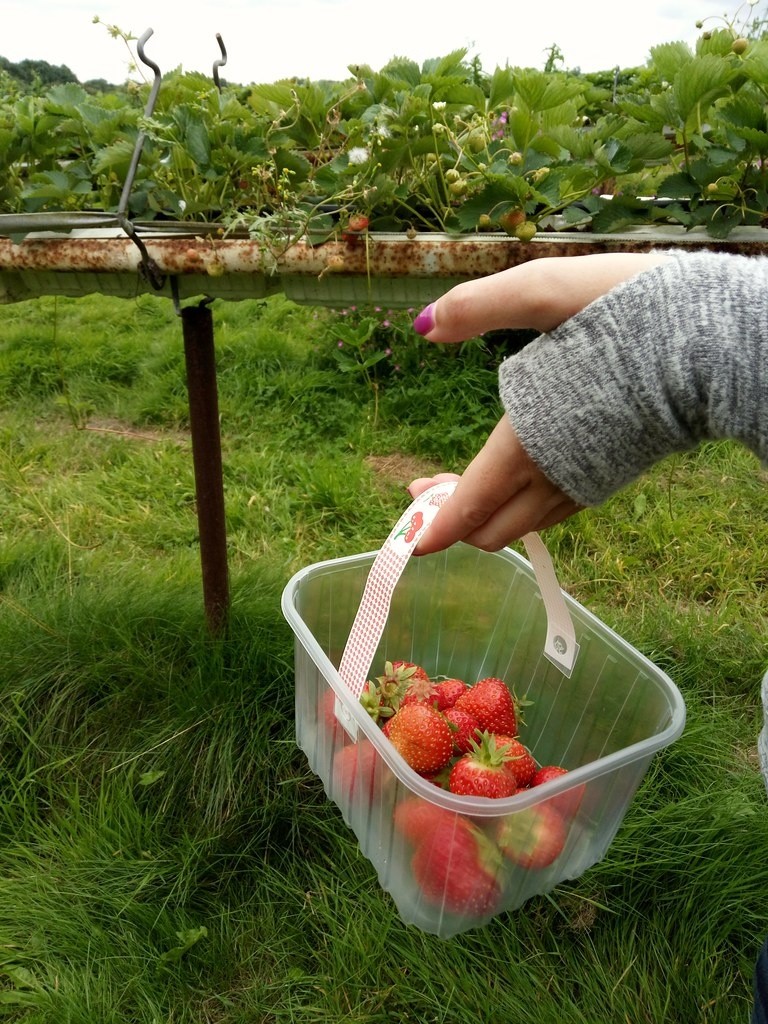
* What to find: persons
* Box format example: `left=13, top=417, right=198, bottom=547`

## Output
left=405, top=250, right=768, bottom=813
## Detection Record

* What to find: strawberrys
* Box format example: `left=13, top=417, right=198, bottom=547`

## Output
left=319, top=658, right=585, bottom=920
left=340, top=205, right=538, bottom=244
